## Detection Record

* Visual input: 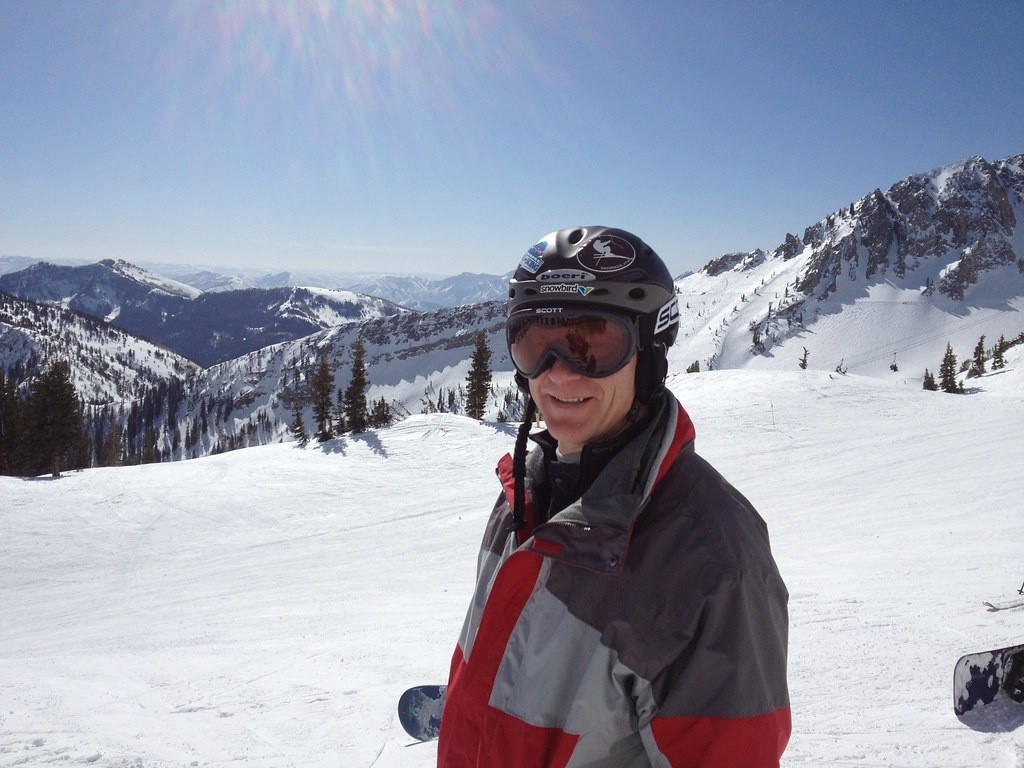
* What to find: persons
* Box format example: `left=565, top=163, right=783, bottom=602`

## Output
left=436, top=225, right=793, bottom=768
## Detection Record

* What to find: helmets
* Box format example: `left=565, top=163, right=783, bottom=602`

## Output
left=506, top=224, right=679, bottom=349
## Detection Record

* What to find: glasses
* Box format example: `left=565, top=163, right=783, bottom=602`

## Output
left=504, top=308, right=647, bottom=380
